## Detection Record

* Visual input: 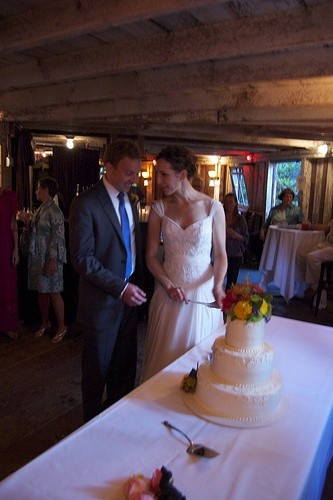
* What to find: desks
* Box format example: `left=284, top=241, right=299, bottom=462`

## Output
left=259, top=224, right=324, bottom=308
left=0, top=316, right=333, bottom=500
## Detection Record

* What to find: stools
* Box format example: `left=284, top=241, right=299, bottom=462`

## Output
left=310, top=260, right=333, bottom=317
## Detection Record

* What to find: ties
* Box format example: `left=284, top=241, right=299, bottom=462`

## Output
left=117, top=192, right=132, bottom=279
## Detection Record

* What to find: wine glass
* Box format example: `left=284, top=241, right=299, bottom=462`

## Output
left=20, top=206, right=33, bottom=231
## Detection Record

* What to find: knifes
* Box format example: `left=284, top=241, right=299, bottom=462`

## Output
left=188, top=300, right=215, bottom=305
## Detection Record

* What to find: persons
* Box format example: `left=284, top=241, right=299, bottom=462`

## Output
left=261, top=187, right=302, bottom=240
left=23, top=179, right=67, bottom=343
left=139, top=146, right=228, bottom=386
left=0, top=188, right=19, bottom=338
left=69, top=140, right=146, bottom=425
left=190, top=179, right=203, bottom=192
left=212, top=192, right=248, bottom=293
left=303, top=220, right=333, bottom=299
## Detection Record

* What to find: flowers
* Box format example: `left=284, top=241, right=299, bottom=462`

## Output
left=180, top=360, right=200, bottom=393
left=122, top=465, right=187, bottom=500
left=220, top=273, right=274, bottom=325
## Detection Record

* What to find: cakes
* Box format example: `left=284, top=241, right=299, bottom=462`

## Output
left=195, top=281, right=283, bottom=422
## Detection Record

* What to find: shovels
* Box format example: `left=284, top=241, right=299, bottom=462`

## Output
left=164, top=421, right=220, bottom=460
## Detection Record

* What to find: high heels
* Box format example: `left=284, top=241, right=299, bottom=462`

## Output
left=34, top=320, right=51, bottom=337
left=51, top=325, right=68, bottom=343
